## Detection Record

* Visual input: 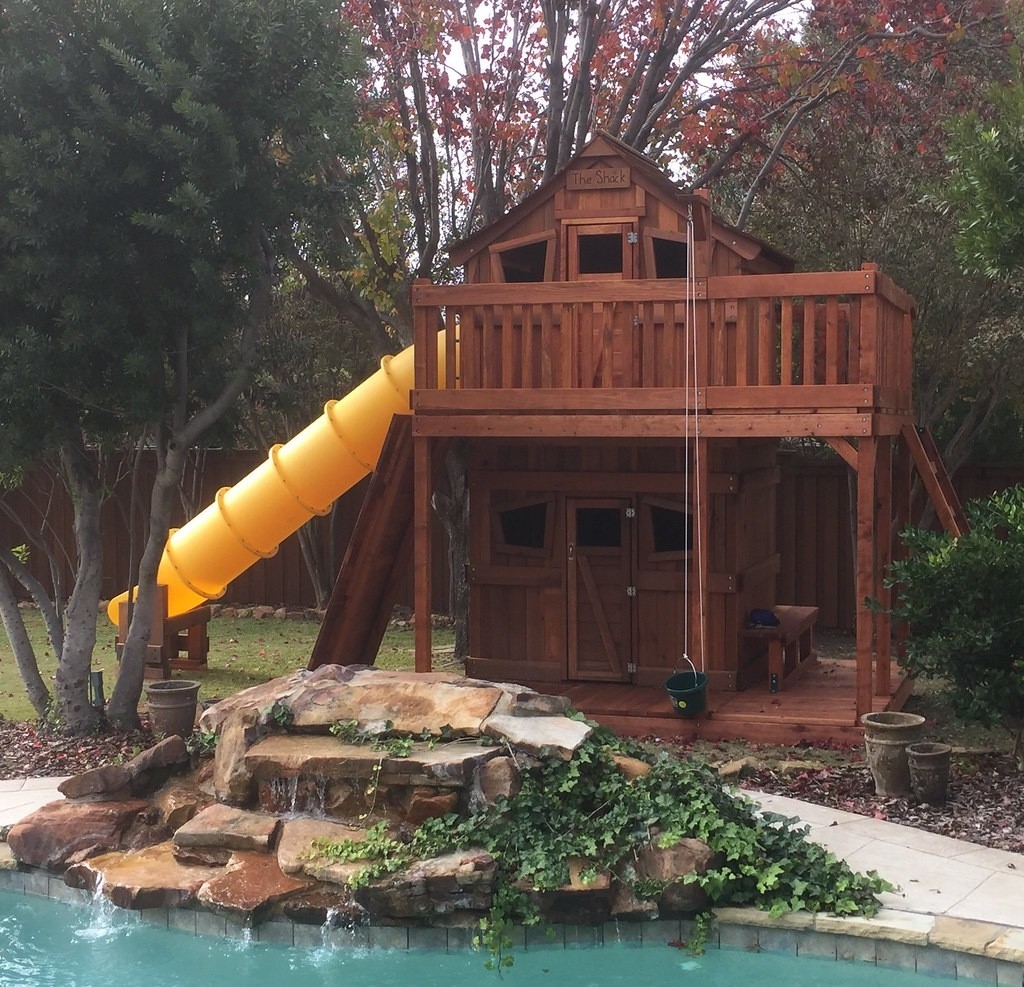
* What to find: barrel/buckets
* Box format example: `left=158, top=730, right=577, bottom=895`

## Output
left=664, top=656, right=708, bottom=717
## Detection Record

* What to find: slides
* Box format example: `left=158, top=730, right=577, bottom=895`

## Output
left=104, top=321, right=463, bottom=630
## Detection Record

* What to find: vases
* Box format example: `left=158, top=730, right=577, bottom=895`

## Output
left=861, top=711, right=925, bottom=798
left=905, top=742, right=952, bottom=806
left=143, top=679, right=201, bottom=742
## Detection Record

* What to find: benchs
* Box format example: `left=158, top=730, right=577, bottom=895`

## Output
left=743, top=605, right=819, bottom=694
